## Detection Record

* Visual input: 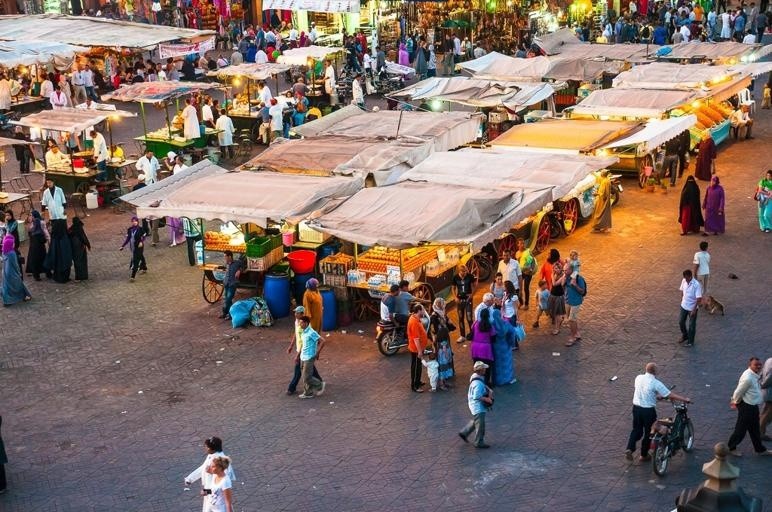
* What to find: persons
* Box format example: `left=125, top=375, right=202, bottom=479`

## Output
left=458, top=362, right=493, bottom=449
left=184, top=437, right=236, bottom=485
left=702, top=176, right=725, bottom=236
left=759, top=358, right=772, bottom=441
left=678, top=175, right=704, bottom=236
left=755, top=170, right=772, bottom=233
left=1, top=0, right=380, bottom=321
left=590, top=169, right=612, bottom=232
left=678, top=270, right=703, bottom=347
left=298, top=316, right=326, bottom=398
left=731, top=105, right=755, bottom=141
left=661, top=129, right=716, bottom=186
left=0, top=415, right=8, bottom=494
left=625, top=362, right=690, bottom=461
left=303, top=278, right=323, bottom=335
left=728, top=357, right=772, bottom=457
left=286, top=306, right=305, bottom=394
left=381, top=239, right=585, bottom=392
left=201, top=457, right=232, bottom=512
left=379, top=1, right=768, bottom=109
left=692, top=242, right=711, bottom=308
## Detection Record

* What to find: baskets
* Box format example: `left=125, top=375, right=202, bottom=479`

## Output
left=319, top=254, right=355, bottom=287
left=246, top=232, right=284, bottom=271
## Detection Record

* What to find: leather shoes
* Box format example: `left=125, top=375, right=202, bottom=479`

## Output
left=416, top=380, right=426, bottom=394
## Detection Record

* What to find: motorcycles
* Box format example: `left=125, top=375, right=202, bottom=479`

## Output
left=356, top=66, right=405, bottom=98
left=649, top=398, right=694, bottom=478
left=375, top=299, right=436, bottom=358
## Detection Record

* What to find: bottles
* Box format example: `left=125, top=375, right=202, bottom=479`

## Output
left=347, top=268, right=366, bottom=285
left=367, top=274, right=386, bottom=287
left=323, top=260, right=355, bottom=275
left=387, top=266, right=401, bottom=289
left=403, top=271, right=416, bottom=286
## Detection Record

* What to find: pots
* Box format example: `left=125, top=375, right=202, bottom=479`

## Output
left=72, top=151, right=93, bottom=158
left=250, top=99, right=260, bottom=105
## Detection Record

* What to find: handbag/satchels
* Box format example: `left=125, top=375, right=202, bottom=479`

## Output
left=513, top=323, right=526, bottom=341
left=483, top=392, right=494, bottom=407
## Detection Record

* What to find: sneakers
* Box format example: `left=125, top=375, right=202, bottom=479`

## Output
left=286, top=381, right=326, bottom=399
left=456, top=336, right=465, bottom=343
left=459, top=433, right=469, bottom=443
left=475, top=445, right=490, bottom=448
left=518, top=304, right=581, bottom=347
left=729, top=433, right=772, bottom=457
left=639, top=455, right=651, bottom=462
left=624, top=450, right=634, bottom=462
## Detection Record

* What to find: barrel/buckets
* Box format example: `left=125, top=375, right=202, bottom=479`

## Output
left=86, top=190, right=98, bottom=209
left=319, top=290, right=336, bottom=331
left=264, top=271, right=290, bottom=319
left=294, top=273, right=314, bottom=304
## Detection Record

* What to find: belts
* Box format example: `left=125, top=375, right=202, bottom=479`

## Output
left=73, top=82, right=84, bottom=88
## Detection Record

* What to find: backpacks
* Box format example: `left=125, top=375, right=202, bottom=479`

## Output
left=576, top=275, right=586, bottom=296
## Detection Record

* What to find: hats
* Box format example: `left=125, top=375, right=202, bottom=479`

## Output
left=293, top=306, right=304, bottom=313
left=474, top=361, right=489, bottom=372
